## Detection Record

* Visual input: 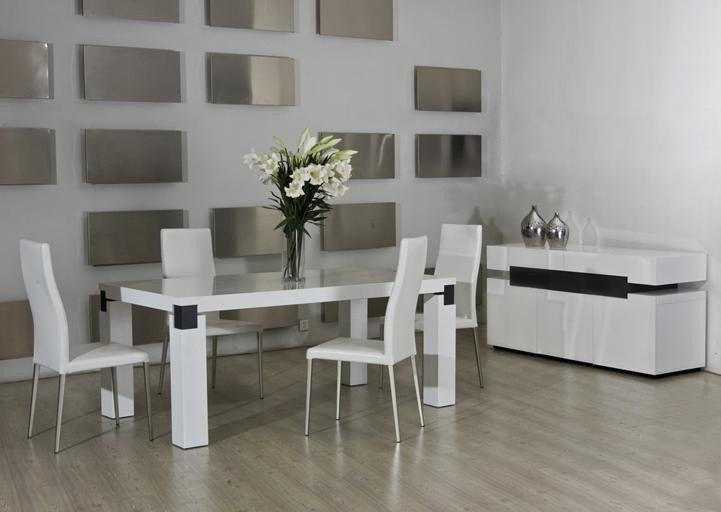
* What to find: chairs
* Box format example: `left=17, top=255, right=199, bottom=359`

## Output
left=378, top=223, right=485, bottom=390
left=305, top=234, right=429, bottom=443
left=19, top=240, right=156, bottom=455
left=159, top=229, right=267, bottom=401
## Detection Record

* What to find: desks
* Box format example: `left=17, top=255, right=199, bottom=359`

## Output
left=483, top=243, right=708, bottom=378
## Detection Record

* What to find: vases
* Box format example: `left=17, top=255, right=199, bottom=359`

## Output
left=280, top=222, right=308, bottom=283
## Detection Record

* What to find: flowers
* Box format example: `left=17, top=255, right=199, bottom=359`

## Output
left=239, top=124, right=361, bottom=240
